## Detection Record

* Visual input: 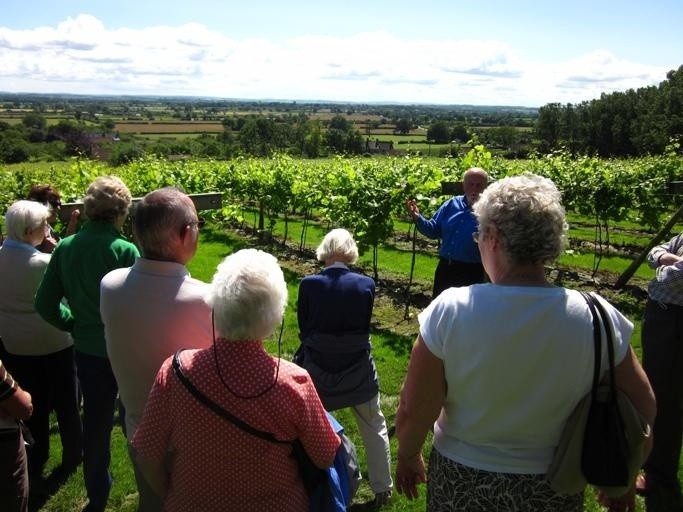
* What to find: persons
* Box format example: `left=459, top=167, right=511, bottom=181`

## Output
left=99, top=187, right=218, bottom=511
left=27, top=185, right=81, bottom=254
left=296, top=228, right=393, bottom=505
left=0, top=199, right=82, bottom=467
left=0, top=359, right=32, bottom=512
left=405, top=168, right=491, bottom=299
left=33, top=175, right=140, bottom=511
left=395, top=171, right=657, bottom=511
left=130, top=247, right=342, bottom=512
left=636, top=230, right=683, bottom=511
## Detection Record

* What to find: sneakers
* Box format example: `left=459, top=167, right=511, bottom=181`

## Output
left=374, top=490, right=391, bottom=505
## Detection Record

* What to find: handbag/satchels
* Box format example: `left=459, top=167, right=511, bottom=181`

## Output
left=579, top=289, right=631, bottom=489
left=171, top=347, right=363, bottom=508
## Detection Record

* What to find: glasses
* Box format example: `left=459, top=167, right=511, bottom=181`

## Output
left=184, top=217, right=206, bottom=228
left=49, top=200, right=61, bottom=209
left=41, top=223, right=50, bottom=229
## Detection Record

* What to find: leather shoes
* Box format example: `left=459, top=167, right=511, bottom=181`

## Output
left=635, top=473, right=647, bottom=493
